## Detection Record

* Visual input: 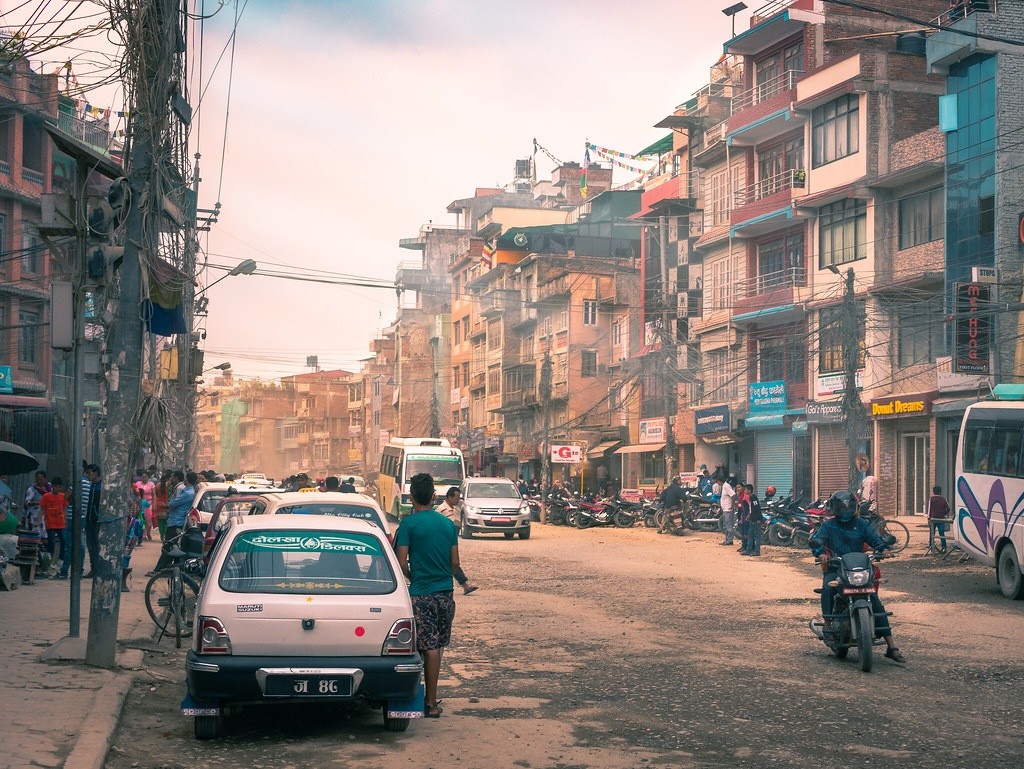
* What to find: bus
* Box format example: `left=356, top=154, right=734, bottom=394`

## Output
left=378, top=437, right=466, bottom=522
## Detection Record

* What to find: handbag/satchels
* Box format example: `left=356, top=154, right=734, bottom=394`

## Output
left=944, top=523, right=950, bottom=532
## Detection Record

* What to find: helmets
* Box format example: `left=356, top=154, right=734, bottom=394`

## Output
left=830, top=490, right=859, bottom=525
left=671, top=475, right=681, bottom=483
left=766, top=486, right=776, bottom=496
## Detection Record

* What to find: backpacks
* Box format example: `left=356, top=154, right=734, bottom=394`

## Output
left=519, top=480, right=528, bottom=494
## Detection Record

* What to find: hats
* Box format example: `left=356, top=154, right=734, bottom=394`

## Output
left=296, top=473, right=308, bottom=480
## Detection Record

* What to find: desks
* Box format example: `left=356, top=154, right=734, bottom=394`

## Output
left=930, top=518, right=956, bottom=556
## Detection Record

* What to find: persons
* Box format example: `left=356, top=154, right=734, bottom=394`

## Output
left=696, top=468, right=722, bottom=498
left=596, top=462, right=609, bottom=496
left=128, top=464, right=198, bottom=580
left=307, top=476, right=356, bottom=493
left=978, top=446, right=1018, bottom=474
left=279, top=472, right=308, bottom=492
left=396, top=474, right=460, bottom=719
left=509, top=474, right=542, bottom=494
left=196, top=469, right=241, bottom=482
left=434, top=485, right=479, bottom=595
left=24, top=458, right=100, bottom=580
left=809, top=490, right=906, bottom=663
left=927, top=485, right=951, bottom=552
left=657, top=477, right=687, bottom=534
left=713, top=473, right=738, bottom=545
left=735, top=483, right=763, bottom=556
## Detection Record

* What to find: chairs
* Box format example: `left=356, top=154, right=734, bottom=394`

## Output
left=243, top=550, right=287, bottom=587
left=319, top=549, right=360, bottom=581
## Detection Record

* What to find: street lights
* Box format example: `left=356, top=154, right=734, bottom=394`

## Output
left=824, top=261, right=860, bottom=502
left=179, top=256, right=260, bottom=468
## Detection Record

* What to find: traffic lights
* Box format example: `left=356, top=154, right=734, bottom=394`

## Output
left=79, top=194, right=126, bottom=284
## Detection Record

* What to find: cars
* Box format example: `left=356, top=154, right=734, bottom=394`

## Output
left=182, top=482, right=285, bottom=537
left=251, top=492, right=395, bottom=574
left=233, top=473, right=273, bottom=486
left=193, top=512, right=432, bottom=741
left=337, top=475, right=367, bottom=494
left=202, top=496, right=261, bottom=563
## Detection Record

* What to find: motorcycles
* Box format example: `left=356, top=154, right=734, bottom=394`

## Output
left=528, top=487, right=910, bottom=554
left=804, top=533, right=900, bottom=671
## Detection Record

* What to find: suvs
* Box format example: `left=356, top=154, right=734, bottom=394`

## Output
left=458, top=476, right=532, bottom=539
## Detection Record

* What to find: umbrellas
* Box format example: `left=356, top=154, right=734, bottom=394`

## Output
left=0, top=441, right=40, bottom=476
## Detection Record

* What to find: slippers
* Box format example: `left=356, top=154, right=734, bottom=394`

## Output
left=462, top=585, right=479, bottom=595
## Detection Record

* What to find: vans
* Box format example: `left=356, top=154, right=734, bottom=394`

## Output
left=953, top=384, right=1024, bottom=601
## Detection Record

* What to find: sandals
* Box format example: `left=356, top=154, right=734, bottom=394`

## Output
left=884, top=648, right=906, bottom=663
left=824, top=618, right=831, bottom=630
left=423, top=700, right=443, bottom=718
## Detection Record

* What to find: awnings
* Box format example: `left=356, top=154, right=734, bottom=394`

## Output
left=613, top=444, right=666, bottom=454
left=587, top=440, right=620, bottom=459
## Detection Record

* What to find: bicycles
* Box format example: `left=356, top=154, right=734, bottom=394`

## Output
left=143, top=525, right=202, bottom=650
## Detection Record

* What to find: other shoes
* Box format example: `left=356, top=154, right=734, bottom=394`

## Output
left=83, top=571, right=93, bottom=578
left=656, top=531, right=663, bottom=534
left=941, top=548, right=945, bottom=553
left=49, top=569, right=68, bottom=579
left=719, top=540, right=760, bottom=556
left=145, top=571, right=155, bottom=577
